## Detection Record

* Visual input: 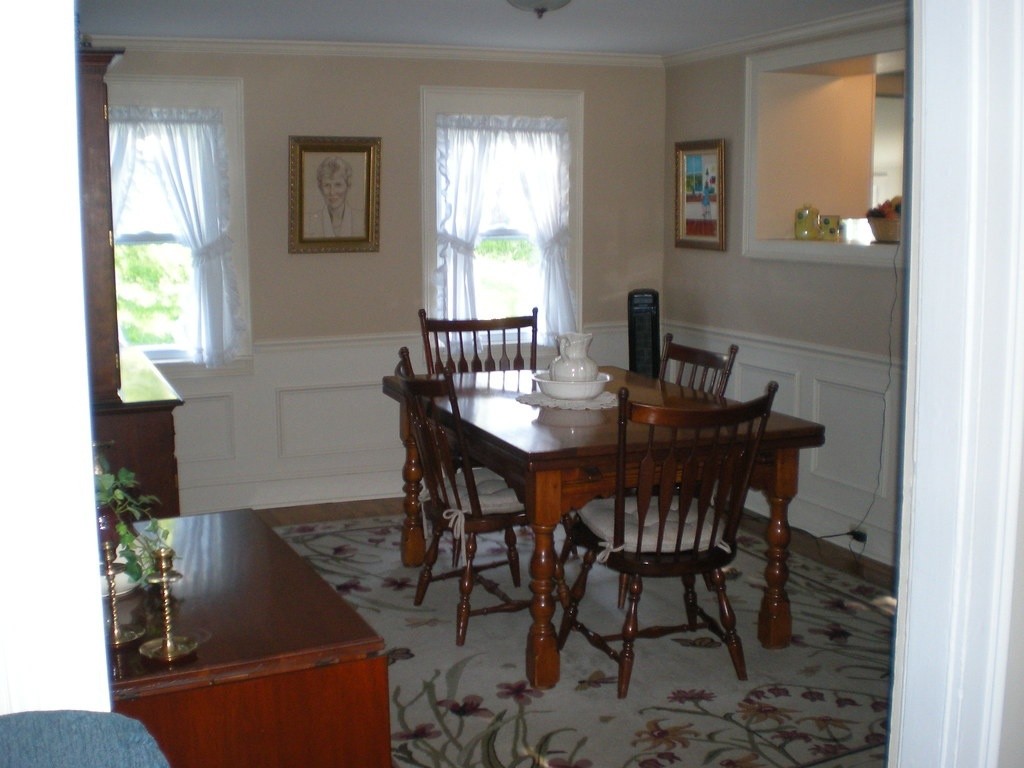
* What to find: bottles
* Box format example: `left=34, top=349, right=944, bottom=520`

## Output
left=794, top=203, right=822, bottom=240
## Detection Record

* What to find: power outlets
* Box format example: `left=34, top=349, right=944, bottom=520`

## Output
left=847, top=525, right=866, bottom=540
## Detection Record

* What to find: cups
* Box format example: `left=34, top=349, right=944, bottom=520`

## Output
left=814, top=214, right=842, bottom=240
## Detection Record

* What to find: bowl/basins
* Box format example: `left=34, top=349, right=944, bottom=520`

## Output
left=531, top=370, right=614, bottom=401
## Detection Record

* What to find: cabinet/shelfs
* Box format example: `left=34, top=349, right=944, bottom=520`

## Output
left=77, top=43, right=184, bottom=515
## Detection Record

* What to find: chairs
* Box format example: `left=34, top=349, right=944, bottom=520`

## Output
left=659, top=334, right=738, bottom=395
left=418, top=306, right=539, bottom=564
left=393, top=347, right=569, bottom=645
left=554, top=381, right=779, bottom=698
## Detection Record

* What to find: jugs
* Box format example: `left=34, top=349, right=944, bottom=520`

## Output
left=549, top=331, right=599, bottom=381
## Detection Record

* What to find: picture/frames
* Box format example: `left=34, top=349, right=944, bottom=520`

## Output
left=673, top=138, right=726, bottom=251
left=288, top=135, right=382, bottom=253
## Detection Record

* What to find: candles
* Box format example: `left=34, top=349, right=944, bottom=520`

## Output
left=96, top=468, right=180, bottom=629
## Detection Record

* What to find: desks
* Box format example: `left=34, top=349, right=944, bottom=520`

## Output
left=97, top=509, right=391, bottom=768
left=382, top=365, right=824, bottom=688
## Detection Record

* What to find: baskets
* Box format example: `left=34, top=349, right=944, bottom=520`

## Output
left=866, top=216, right=901, bottom=244
